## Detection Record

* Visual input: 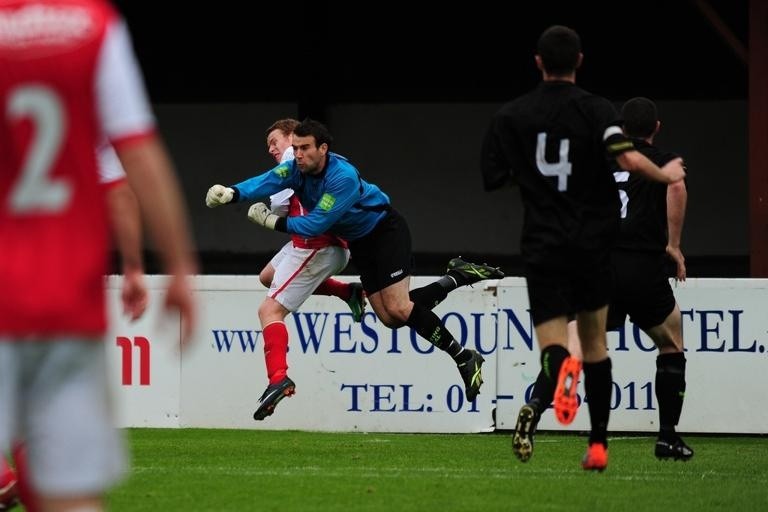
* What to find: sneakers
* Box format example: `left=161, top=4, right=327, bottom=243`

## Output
left=655, top=435, right=693, bottom=461
left=554, top=357, right=582, bottom=424
left=346, top=282, right=367, bottom=321
left=457, top=350, right=485, bottom=402
left=582, top=442, right=608, bottom=472
left=254, top=375, right=295, bottom=420
left=512, top=403, right=540, bottom=462
left=447, top=256, right=505, bottom=284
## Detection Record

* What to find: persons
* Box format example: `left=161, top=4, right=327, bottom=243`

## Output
left=200, top=120, right=506, bottom=403
left=1, top=3, right=199, bottom=512
left=547, top=93, right=700, bottom=464
left=0, top=130, right=153, bottom=510
left=242, top=114, right=371, bottom=423
left=477, top=22, right=690, bottom=476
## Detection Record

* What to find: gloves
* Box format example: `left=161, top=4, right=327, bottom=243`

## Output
left=247, top=202, right=279, bottom=231
left=205, top=185, right=235, bottom=209
